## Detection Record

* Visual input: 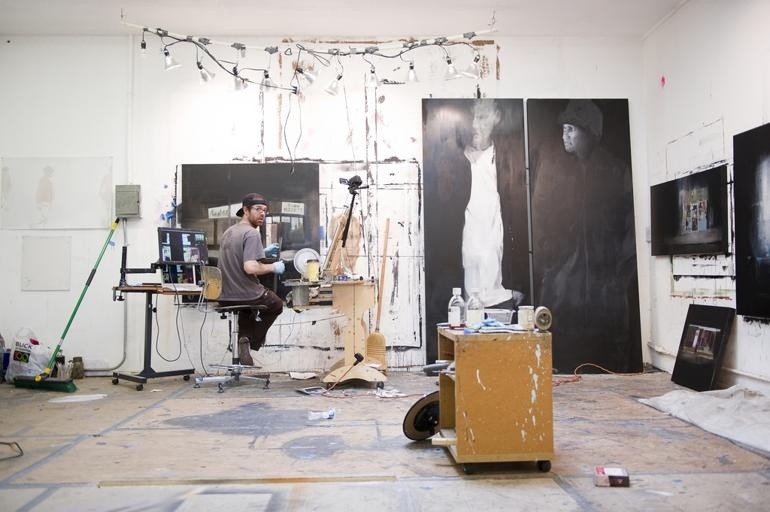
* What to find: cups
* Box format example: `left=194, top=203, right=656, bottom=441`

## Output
left=306, top=258, right=321, bottom=283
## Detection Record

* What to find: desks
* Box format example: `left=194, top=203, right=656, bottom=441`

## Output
left=113, top=286, right=203, bottom=394
left=318, top=279, right=387, bottom=391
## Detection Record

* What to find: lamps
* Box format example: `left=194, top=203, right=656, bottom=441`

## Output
left=158, top=30, right=487, bottom=95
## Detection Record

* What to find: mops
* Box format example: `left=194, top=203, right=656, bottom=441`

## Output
left=14, top=219, right=119, bottom=393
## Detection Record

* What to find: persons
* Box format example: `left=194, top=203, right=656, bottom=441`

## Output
left=426, top=99, right=527, bottom=309
left=216, top=192, right=286, bottom=366
left=529, top=100, right=636, bottom=374
left=689, top=329, right=702, bottom=353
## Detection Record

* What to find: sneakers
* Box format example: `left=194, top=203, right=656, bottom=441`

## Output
left=238, top=335, right=253, bottom=366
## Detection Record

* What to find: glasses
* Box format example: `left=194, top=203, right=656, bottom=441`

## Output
left=250, top=206, right=267, bottom=213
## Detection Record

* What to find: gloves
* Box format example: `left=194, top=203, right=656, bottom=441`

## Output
left=264, top=242, right=280, bottom=257
left=273, top=260, right=286, bottom=275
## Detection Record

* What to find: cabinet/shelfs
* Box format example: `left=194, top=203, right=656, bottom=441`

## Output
left=436, top=326, right=555, bottom=476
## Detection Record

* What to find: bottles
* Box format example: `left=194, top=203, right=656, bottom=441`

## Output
left=308, top=407, right=342, bottom=421
left=517, top=305, right=535, bottom=331
left=466, top=288, right=485, bottom=330
left=447, top=287, right=467, bottom=329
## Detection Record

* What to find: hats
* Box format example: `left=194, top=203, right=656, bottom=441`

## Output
left=236, top=193, right=268, bottom=218
left=560, top=100, right=605, bottom=144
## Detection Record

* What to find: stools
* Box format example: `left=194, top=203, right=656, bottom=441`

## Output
left=194, top=305, right=270, bottom=394
